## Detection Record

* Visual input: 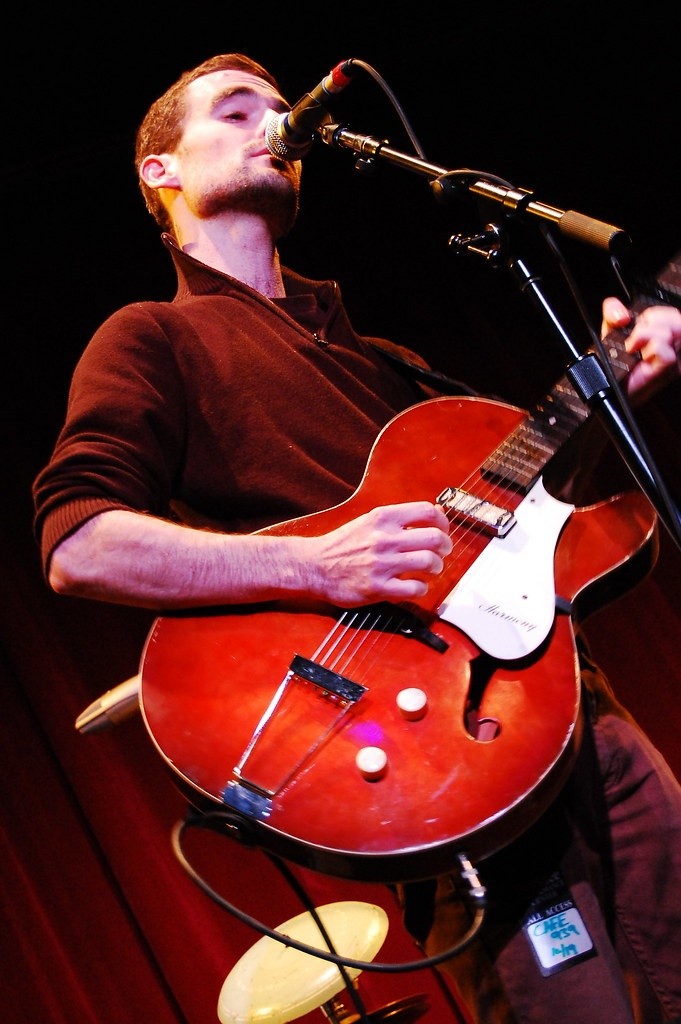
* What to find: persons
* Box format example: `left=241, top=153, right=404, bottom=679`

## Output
left=33, top=51, right=681, bottom=1024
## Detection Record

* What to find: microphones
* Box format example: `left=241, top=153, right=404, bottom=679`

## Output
left=266, top=59, right=361, bottom=162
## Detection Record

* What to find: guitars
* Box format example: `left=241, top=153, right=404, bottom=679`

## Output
left=119, top=253, right=681, bottom=882
